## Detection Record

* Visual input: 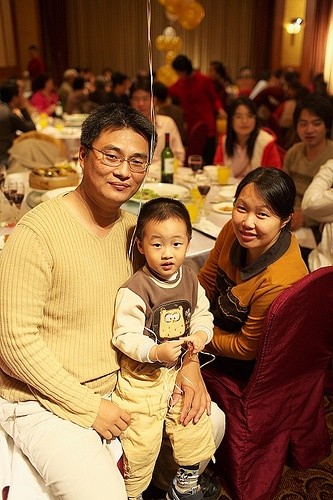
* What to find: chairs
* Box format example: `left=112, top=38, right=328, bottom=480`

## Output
left=5, top=132, right=67, bottom=174
left=199, top=265, right=333, bottom=500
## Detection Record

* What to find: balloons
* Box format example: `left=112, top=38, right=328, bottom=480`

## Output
left=155, top=26, right=183, bottom=85
left=159, top=0, right=205, bottom=30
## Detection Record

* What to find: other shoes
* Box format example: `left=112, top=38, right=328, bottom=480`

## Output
left=198, top=472, right=222, bottom=498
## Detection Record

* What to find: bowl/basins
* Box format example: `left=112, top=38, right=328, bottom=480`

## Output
left=218, top=191, right=235, bottom=201
left=203, top=166, right=218, bottom=181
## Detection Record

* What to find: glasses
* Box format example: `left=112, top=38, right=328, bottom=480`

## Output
left=88, top=145, right=151, bottom=173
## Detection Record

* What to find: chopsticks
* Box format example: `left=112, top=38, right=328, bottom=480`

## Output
left=192, top=227, right=217, bottom=241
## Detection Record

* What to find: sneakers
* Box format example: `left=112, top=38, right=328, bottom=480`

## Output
left=167, top=484, right=205, bottom=500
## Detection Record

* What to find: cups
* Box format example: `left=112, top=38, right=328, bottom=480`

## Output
left=173, top=158, right=178, bottom=174
left=184, top=187, right=205, bottom=224
left=217, top=162, right=231, bottom=185
left=31, top=112, right=63, bottom=132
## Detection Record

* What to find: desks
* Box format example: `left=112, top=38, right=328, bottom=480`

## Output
left=42, top=125, right=83, bottom=160
left=0, top=165, right=240, bottom=277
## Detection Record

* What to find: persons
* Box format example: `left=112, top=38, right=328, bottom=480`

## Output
left=0, top=82, right=37, bottom=166
left=165, top=56, right=229, bottom=164
left=101, top=72, right=130, bottom=106
left=127, top=83, right=186, bottom=170
left=29, top=75, right=61, bottom=116
left=249, top=69, right=328, bottom=137
left=283, top=95, right=333, bottom=208
left=208, top=62, right=233, bottom=101
left=148, top=83, right=186, bottom=141
left=300, top=159, right=333, bottom=271
left=215, top=97, right=281, bottom=176
left=112, top=198, right=217, bottom=499
left=0, top=103, right=211, bottom=500
left=23, top=45, right=47, bottom=79
left=197, top=167, right=309, bottom=380
left=60, top=67, right=113, bottom=113
left=9, top=75, right=33, bottom=116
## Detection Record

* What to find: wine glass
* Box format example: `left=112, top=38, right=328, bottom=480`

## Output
left=188, top=155, right=202, bottom=177
left=0, top=163, right=24, bottom=210
left=198, top=178, right=211, bottom=217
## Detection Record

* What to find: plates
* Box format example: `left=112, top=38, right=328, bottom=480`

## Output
left=128, top=183, right=190, bottom=204
left=0, top=234, right=11, bottom=249
left=63, top=113, right=92, bottom=126
left=41, top=186, right=81, bottom=201
left=222, top=186, right=238, bottom=191
left=212, top=201, right=234, bottom=215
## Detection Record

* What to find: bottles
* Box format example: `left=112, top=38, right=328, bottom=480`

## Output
left=160, top=132, right=174, bottom=184
left=55, top=102, right=64, bottom=118
